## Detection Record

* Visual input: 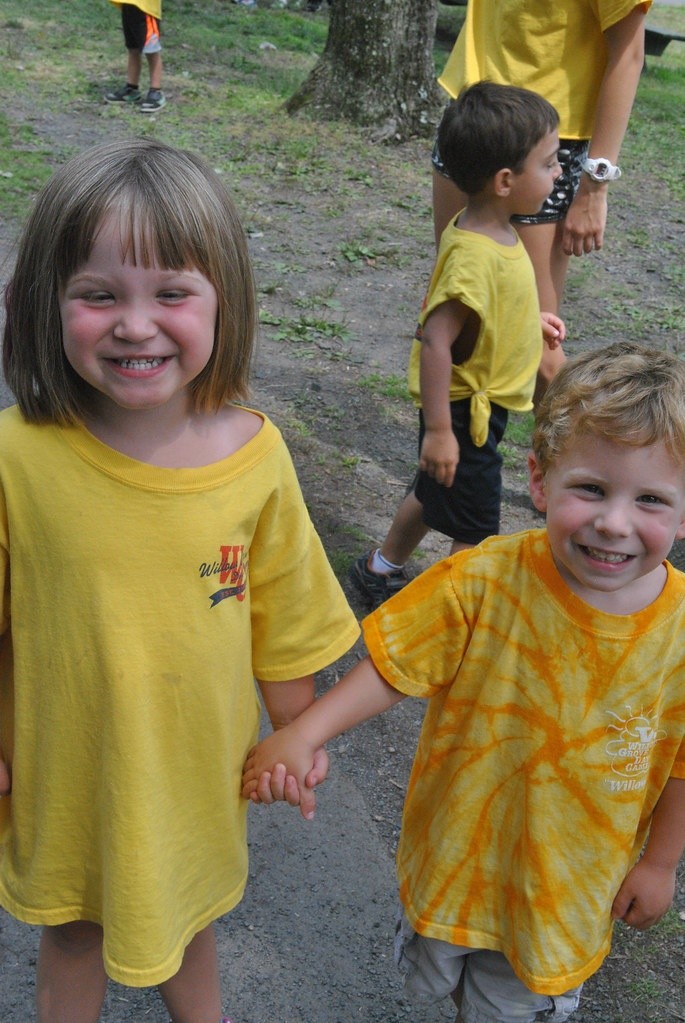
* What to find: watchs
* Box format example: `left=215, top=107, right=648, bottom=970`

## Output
left=582, top=157, right=621, bottom=183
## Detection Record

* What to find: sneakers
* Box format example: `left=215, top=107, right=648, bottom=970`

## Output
left=350, top=547, right=407, bottom=613
left=140, top=89, right=166, bottom=113
left=104, top=85, right=143, bottom=103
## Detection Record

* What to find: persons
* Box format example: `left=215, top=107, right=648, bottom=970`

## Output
left=431, top=0, right=649, bottom=414
left=0, top=81, right=685, bottom=1023
left=104, top=0, right=168, bottom=112
